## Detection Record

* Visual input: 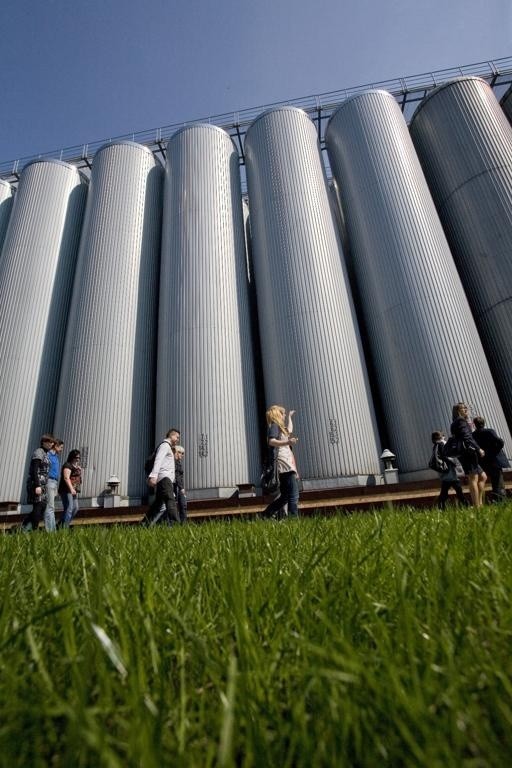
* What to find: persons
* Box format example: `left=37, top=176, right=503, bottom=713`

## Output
left=427, top=429, right=469, bottom=510
left=43, top=438, right=65, bottom=532
left=266, top=406, right=293, bottom=521
left=136, top=428, right=181, bottom=528
left=57, top=448, right=84, bottom=528
left=175, top=445, right=187, bottom=525
left=471, top=416, right=505, bottom=504
left=23, top=432, right=57, bottom=532
left=151, top=446, right=179, bottom=528
left=450, top=402, right=488, bottom=508
left=260, top=403, right=300, bottom=521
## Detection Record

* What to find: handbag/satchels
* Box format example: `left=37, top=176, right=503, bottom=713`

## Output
left=296, top=474, right=303, bottom=491
left=261, top=476, right=280, bottom=497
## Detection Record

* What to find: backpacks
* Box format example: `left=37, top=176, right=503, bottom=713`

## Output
left=428, top=437, right=464, bottom=474
left=145, top=452, right=155, bottom=475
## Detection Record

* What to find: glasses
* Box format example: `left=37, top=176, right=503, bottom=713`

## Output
left=74, top=457, right=81, bottom=459
